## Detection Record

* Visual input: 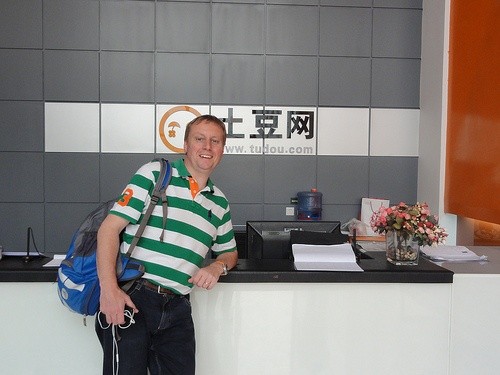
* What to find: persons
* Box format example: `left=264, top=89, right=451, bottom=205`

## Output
left=95, top=115, right=238, bottom=375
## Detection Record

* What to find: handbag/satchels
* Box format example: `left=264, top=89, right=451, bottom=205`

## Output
left=342, top=219, right=367, bottom=235
left=361, top=198, right=389, bottom=236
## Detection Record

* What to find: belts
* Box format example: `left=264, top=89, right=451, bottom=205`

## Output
left=137, top=280, right=170, bottom=300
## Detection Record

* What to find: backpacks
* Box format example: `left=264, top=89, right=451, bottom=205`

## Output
left=58, top=157, right=172, bottom=327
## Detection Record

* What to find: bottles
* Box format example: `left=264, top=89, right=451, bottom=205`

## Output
left=297, top=191, right=323, bottom=220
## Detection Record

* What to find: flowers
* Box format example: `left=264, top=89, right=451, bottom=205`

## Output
left=370, top=201, right=448, bottom=248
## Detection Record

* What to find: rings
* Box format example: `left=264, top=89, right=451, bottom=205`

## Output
left=204, top=282, right=208, bottom=284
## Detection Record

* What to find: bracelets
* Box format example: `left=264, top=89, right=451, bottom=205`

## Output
left=214, top=260, right=229, bottom=276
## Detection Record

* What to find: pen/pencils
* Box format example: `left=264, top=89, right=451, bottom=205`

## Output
left=352, top=228, right=356, bottom=259
left=26, top=227, right=32, bottom=255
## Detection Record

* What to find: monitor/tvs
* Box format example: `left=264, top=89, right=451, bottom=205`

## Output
left=246, top=221, right=341, bottom=262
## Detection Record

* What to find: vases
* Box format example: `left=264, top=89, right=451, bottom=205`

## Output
left=385, top=229, right=420, bottom=265
